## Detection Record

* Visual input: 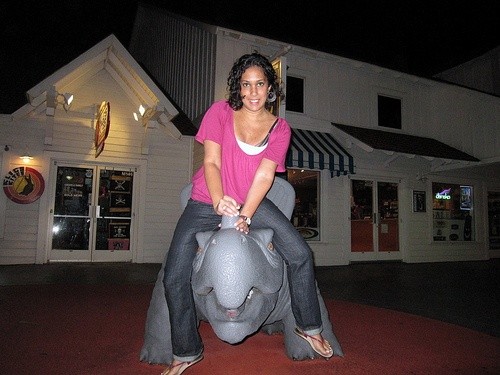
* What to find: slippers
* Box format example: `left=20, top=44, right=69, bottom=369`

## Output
left=292, top=327, right=333, bottom=358
left=161, top=356, right=204, bottom=375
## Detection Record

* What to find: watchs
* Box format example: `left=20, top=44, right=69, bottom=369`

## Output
left=240, top=215, right=251, bottom=226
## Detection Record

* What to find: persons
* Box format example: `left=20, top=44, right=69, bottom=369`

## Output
left=160, top=52, right=334, bottom=375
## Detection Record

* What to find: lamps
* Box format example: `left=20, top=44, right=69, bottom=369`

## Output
left=132, top=112, right=143, bottom=121
left=19, top=152, right=33, bottom=163
left=57, top=92, right=75, bottom=105
left=139, top=103, right=148, bottom=117
left=58, top=101, right=72, bottom=111
